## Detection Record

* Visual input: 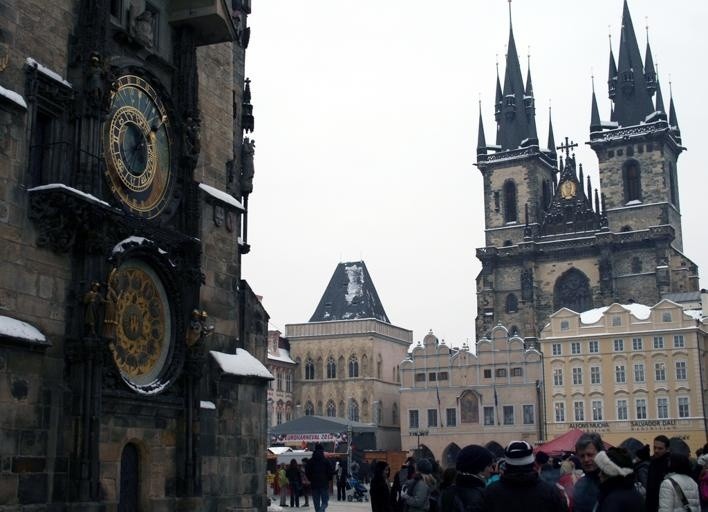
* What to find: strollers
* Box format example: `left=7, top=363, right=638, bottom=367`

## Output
left=346, top=476, right=369, bottom=502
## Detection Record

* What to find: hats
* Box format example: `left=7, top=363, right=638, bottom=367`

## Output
left=594, top=446, right=633, bottom=477
left=456, top=441, right=534, bottom=474
left=536, top=452, right=549, bottom=464
left=417, top=459, right=433, bottom=474
left=636, top=444, right=650, bottom=459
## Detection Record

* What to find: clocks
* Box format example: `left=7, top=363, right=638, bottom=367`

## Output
left=105, top=235, right=188, bottom=398
left=99, top=58, right=187, bottom=223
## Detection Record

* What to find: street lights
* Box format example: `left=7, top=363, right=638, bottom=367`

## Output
left=409, top=429, right=428, bottom=448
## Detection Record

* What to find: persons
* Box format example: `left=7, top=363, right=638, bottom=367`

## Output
left=274, top=443, right=373, bottom=511
left=83, top=280, right=106, bottom=336
left=102, top=277, right=123, bottom=338
left=370, top=431, right=708, bottom=511
left=85, top=57, right=108, bottom=105
left=181, top=117, right=200, bottom=157
left=190, top=309, right=208, bottom=360
